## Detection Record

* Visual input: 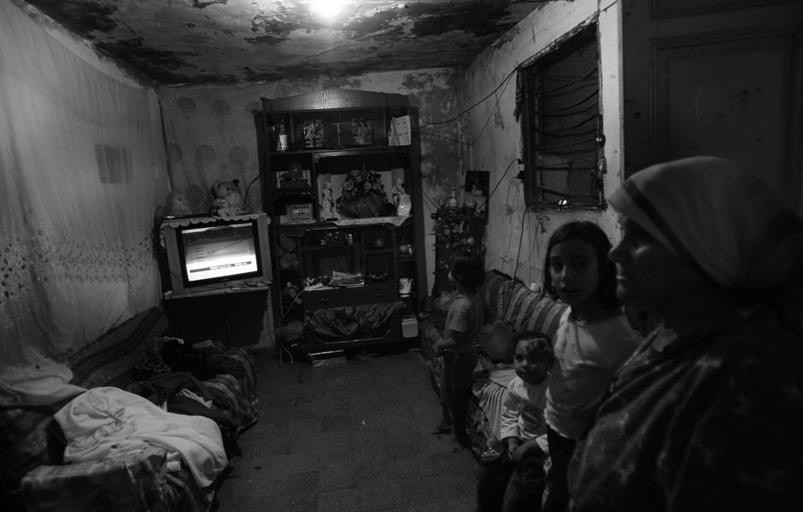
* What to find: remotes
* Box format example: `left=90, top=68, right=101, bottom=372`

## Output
left=263, top=280, right=273, bottom=286
left=247, top=282, right=257, bottom=287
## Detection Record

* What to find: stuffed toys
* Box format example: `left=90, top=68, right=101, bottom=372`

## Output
left=164, top=190, right=191, bottom=218
left=209, top=178, right=244, bottom=217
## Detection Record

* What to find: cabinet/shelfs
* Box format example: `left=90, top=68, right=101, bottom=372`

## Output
left=259, top=88, right=427, bottom=357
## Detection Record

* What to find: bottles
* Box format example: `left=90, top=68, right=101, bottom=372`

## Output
left=277, top=119, right=289, bottom=152
left=447, top=186, right=458, bottom=210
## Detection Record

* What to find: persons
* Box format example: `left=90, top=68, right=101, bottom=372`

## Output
left=569, top=158, right=802, bottom=510
left=429, top=251, right=486, bottom=435
left=476, top=331, right=551, bottom=510
left=538, top=220, right=641, bottom=512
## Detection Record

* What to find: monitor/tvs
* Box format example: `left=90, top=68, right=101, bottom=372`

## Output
left=163, top=213, right=273, bottom=296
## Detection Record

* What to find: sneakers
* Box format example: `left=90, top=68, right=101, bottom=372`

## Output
left=430, top=427, right=466, bottom=453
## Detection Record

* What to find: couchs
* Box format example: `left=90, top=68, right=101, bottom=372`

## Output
left=0, top=306, right=246, bottom=510
left=423, top=267, right=568, bottom=453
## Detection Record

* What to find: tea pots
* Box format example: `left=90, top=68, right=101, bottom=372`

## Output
left=398, top=276, right=413, bottom=298
